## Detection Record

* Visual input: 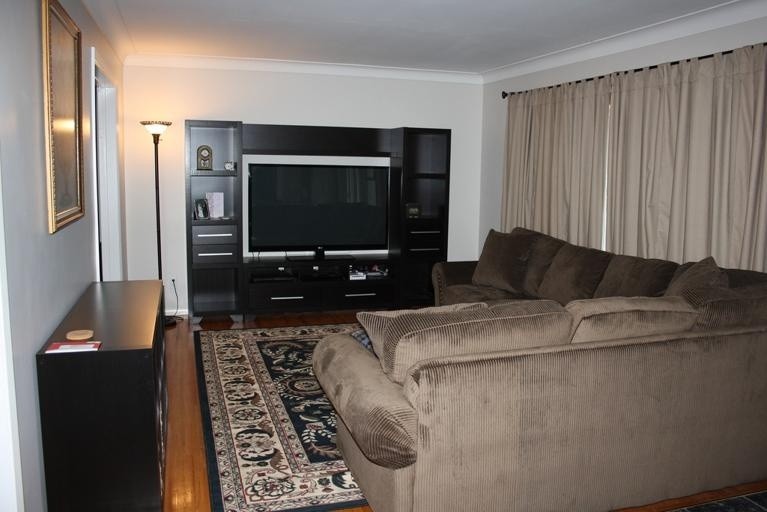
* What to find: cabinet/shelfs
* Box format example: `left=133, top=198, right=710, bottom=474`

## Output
left=36, top=278, right=166, bottom=512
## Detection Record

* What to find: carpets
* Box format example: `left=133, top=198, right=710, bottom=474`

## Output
left=193, top=322, right=369, bottom=512
left=311, top=259, right=767, bottom=512
left=667, top=490, right=767, bottom=511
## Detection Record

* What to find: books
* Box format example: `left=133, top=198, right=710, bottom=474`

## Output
left=45, top=340, right=102, bottom=354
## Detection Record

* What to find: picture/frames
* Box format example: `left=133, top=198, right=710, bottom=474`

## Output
left=39, top=0, right=87, bottom=235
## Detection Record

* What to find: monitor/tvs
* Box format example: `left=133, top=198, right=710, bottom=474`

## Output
left=241, top=155, right=390, bottom=264
